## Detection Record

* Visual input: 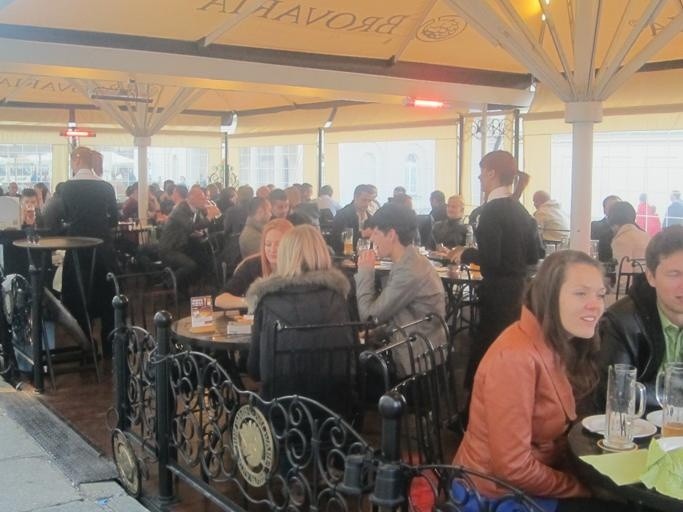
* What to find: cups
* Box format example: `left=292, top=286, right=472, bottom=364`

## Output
left=357, top=238, right=371, bottom=254
left=590, top=240, right=599, bottom=262
left=419, top=246, right=425, bottom=253
left=435, top=243, right=443, bottom=252
left=603, top=364, right=647, bottom=450
left=204, top=200, right=222, bottom=219
left=655, top=362, right=683, bottom=436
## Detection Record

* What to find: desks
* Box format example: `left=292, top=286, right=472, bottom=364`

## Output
left=13, top=237, right=103, bottom=386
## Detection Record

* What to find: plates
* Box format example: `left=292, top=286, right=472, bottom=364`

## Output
left=582, top=414, right=657, bottom=438
left=646, top=407, right=683, bottom=427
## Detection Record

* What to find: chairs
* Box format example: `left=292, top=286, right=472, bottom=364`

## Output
left=114, top=224, right=237, bottom=265
left=543, top=239, right=564, bottom=253
left=107, top=266, right=235, bottom=393
left=614, top=255, right=644, bottom=303
left=269, top=310, right=455, bottom=478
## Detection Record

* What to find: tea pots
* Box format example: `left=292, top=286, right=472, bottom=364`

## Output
left=342, top=228, right=353, bottom=255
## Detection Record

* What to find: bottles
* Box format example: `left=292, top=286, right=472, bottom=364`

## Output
left=465, top=225, right=474, bottom=247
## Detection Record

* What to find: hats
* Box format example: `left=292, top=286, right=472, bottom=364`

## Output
left=364, top=202, right=416, bottom=227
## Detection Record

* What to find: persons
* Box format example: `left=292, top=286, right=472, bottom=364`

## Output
left=637, top=193, right=661, bottom=235
left=449, top=151, right=544, bottom=432
left=451, top=248, right=603, bottom=512
left=0, top=148, right=118, bottom=371
left=534, top=190, right=571, bottom=241
left=663, top=190, right=683, bottom=227
left=590, top=195, right=620, bottom=239
left=591, top=228, right=683, bottom=407
left=599, top=202, right=650, bottom=292
left=119, top=177, right=469, bottom=425
